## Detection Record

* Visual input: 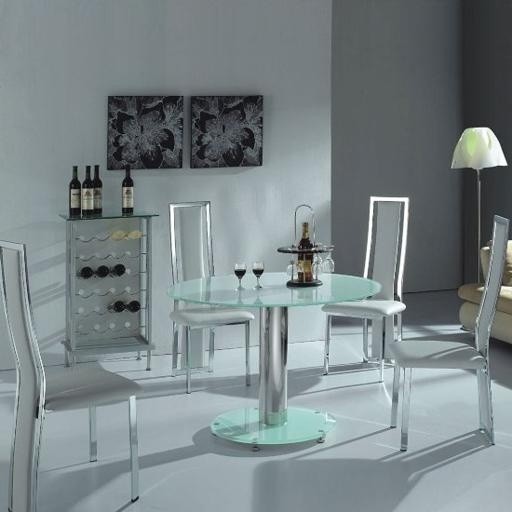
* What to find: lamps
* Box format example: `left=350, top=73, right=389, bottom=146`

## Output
left=451, top=127, right=507, bottom=284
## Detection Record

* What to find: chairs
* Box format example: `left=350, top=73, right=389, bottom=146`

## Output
left=322, top=196, right=410, bottom=375
left=169, top=201, right=254, bottom=395
left=458, top=240, right=512, bottom=344
left=0, top=241, right=139, bottom=512
left=390, top=214, right=509, bottom=452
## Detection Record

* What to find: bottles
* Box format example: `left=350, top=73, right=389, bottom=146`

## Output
left=66, top=163, right=103, bottom=219
left=107, top=299, right=140, bottom=314
left=79, top=261, right=126, bottom=279
left=120, top=164, right=134, bottom=216
left=296, top=222, right=315, bottom=284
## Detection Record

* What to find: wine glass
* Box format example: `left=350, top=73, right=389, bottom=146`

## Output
left=236, top=291, right=245, bottom=306
left=254, top=291, right=262, bottom=305
left=285, top=244, right=337, bottom=282
left=252, top=261, right=265, bottom=289
left=233, top=263, right=246, bottom=292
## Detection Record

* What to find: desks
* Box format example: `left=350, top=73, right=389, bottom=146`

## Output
left=169, top=272, right=381, bottom=452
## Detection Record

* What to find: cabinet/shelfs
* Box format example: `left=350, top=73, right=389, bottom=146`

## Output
left=59, top=212, right=159, bottom=371
